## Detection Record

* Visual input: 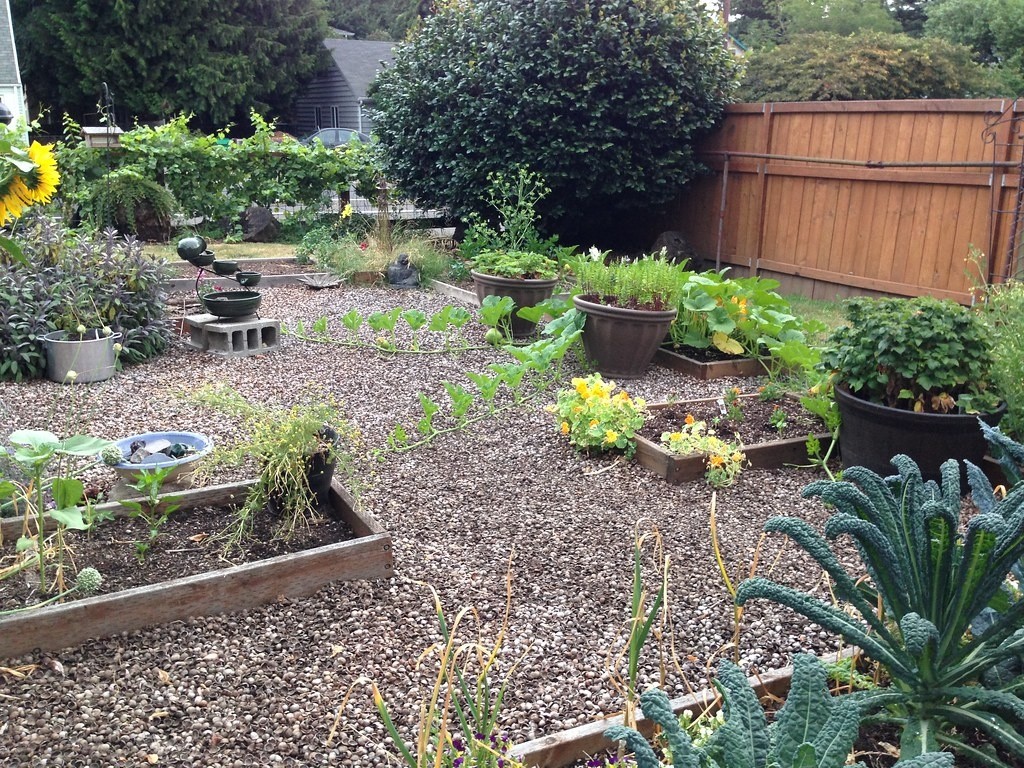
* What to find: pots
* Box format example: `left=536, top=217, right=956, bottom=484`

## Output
left=36, top=329, right=122, bottom=383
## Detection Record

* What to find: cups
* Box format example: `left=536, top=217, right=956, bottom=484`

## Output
left=177, top=235, right=207, bottom=260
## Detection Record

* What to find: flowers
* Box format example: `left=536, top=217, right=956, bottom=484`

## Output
left=175, top=384, right=363, bottom=560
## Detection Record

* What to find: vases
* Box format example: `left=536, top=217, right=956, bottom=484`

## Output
left=267, top=428, right=340, bottom=514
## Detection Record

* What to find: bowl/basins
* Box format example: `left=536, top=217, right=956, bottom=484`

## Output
left=203, top=291, right=261, bottom=316
left=190, top=250, right=215, bottom=266
left=236, top=272, right=261, bottom=285
left=101, top=432, right=211, bottom=483
left=213, top=260, right=237, bottom=275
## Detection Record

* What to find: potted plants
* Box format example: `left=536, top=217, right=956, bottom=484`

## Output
left=576, top=246, right=677, bottom=379
left=818, top=295, right=1003, bottom=487
left=470, top=250, right=558, bottom=337
